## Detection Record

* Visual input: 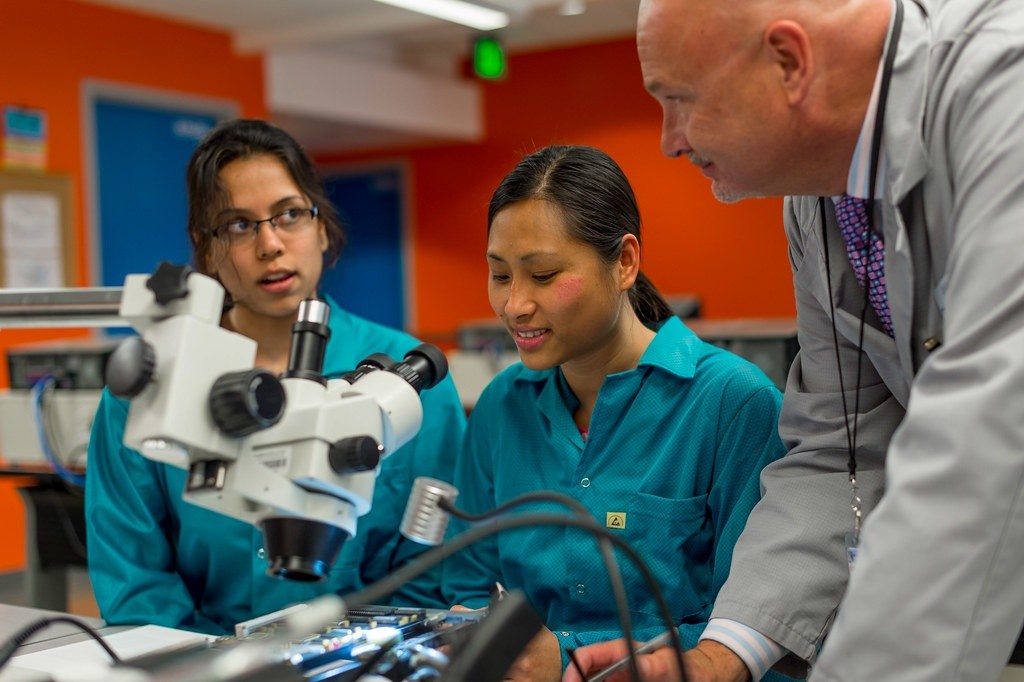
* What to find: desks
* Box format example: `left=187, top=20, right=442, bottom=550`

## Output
left=685, top=317, right=802, bottom=392
left=0, top=562, right=138, bottom=682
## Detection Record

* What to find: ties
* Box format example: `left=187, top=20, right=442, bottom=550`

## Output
left=834, top=192, right=895, bottom=341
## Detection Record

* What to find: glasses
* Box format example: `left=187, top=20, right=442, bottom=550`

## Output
left=202, top=205, right=319, bottom=249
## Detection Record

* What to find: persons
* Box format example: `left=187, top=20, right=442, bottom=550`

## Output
left=85, top=120, right=472, bottom=644
left=440, top=144, right=793, bottom=682
left=556, top=0, right=1024, bottom=682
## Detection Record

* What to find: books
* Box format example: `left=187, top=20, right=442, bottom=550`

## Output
left=8, top=622, right=312, bottom=682
